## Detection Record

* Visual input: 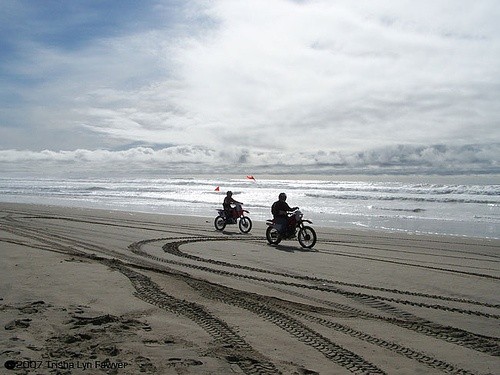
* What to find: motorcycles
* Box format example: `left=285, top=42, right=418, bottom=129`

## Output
left=214, top=202, right=252, bottom=234
left=265, top=209, right=317, bottom=248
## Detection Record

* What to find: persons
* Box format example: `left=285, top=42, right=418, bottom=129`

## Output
left=222, top=191, right=242, bottom=223
left=270, top=193, right=299, bottom=239
